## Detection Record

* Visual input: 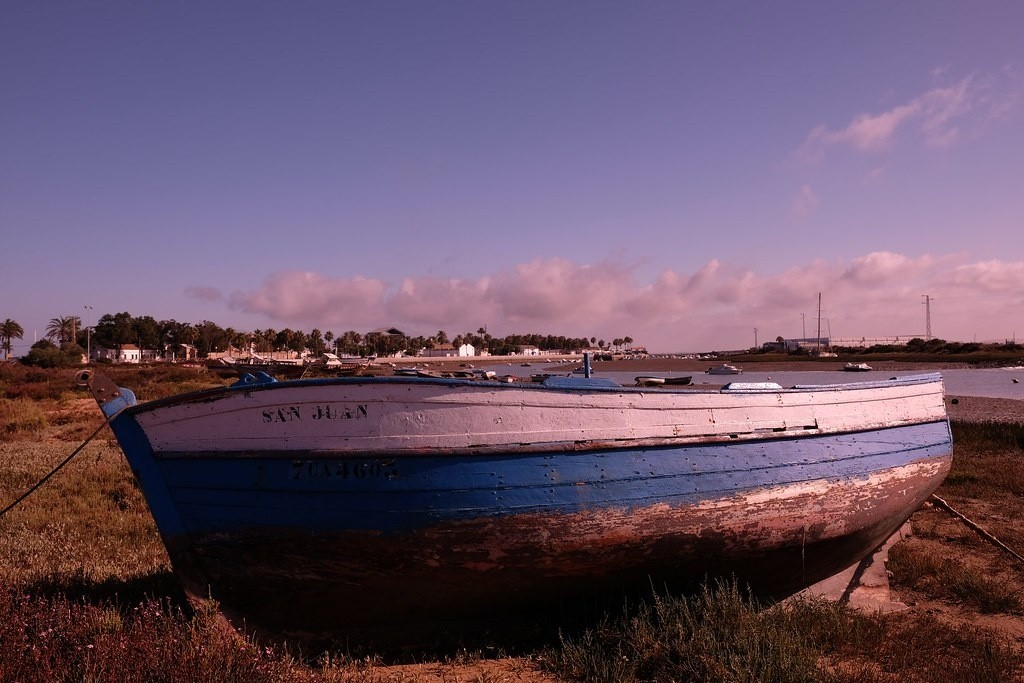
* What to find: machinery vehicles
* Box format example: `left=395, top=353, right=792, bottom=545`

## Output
left=752, top=327, right=759, bottom=346
left=920, top=294, right=935, bottom=341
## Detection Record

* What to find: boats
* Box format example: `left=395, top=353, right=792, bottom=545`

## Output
left=1011, top=378, right=1019, bottom=384
left=413, top=350, right=720, bottom=372
left=633, top=374, right=693, bottom=387
left=481, top=370, right=496, bottom=380
left=704, top=364, right=742, bottom=375
left=73, top=369, right=957, bottom=603
left=392, top=367, right=423, bottom=376
left=529, top=373, right=552, bottom=383
left=842, top=360, right=874, bottom=373
left=416, top=368, right=476, bottom=381
left=497, top=376, right=514, bottom=383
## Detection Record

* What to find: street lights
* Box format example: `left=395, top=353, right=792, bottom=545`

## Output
left=83, top=303, right=94, bottom=365
